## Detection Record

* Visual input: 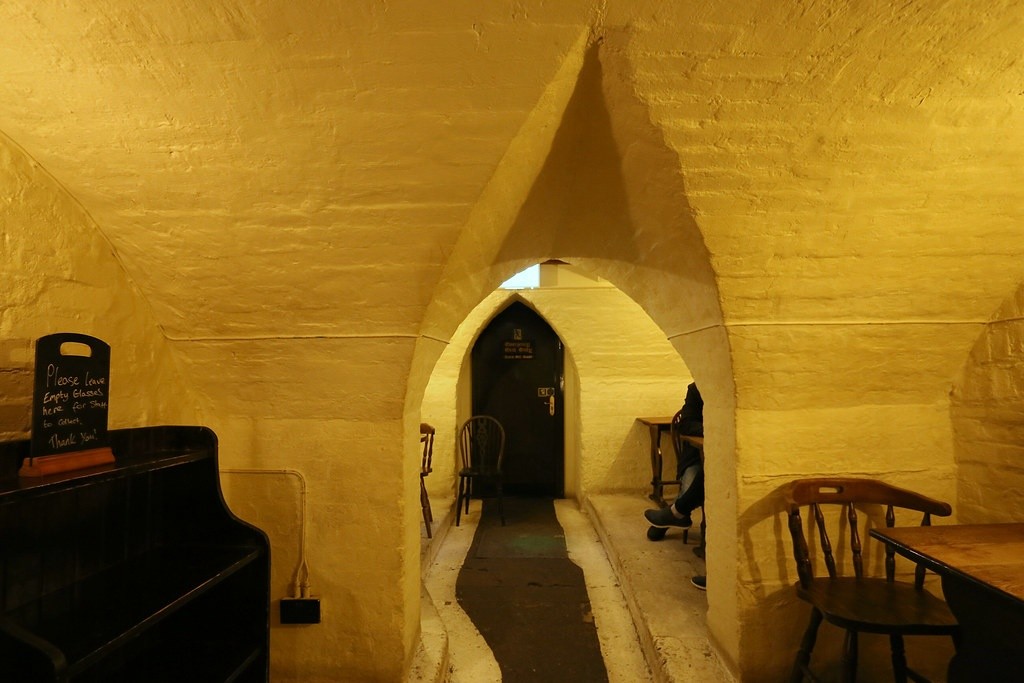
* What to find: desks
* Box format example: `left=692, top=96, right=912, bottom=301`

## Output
left=869, top=520, right=1024, bottom=683
left=680, top=435, right=705, bottom=561
left=638, top=416, right=677, bottom=509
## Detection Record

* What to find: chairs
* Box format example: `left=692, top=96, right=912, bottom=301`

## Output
left=456, top=415, right=507, bottom=527
left=420, top=424, right=435, bottom=539
left=785, top=477, right=958, bottom=683
left=671, top=409, right=688, bottom=544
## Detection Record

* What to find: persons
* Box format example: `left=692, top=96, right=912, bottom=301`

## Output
left=644, top=382, right=706, bottom=591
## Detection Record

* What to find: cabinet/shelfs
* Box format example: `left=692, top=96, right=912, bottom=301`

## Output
left=0, top=424, right=270, bottom=683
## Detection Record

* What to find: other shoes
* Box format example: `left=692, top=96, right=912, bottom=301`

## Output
left=647, top=526, right=669, bottom=541
left=693, top=546, right=701, bottom=557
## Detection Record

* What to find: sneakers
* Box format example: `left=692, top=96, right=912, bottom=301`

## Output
left=690, top=576, right=706, bottom=589
left=643, top=504, right=693, bottom=529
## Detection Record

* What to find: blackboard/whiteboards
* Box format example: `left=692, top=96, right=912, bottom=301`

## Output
left=30, top=333, right=111, bottom=457
